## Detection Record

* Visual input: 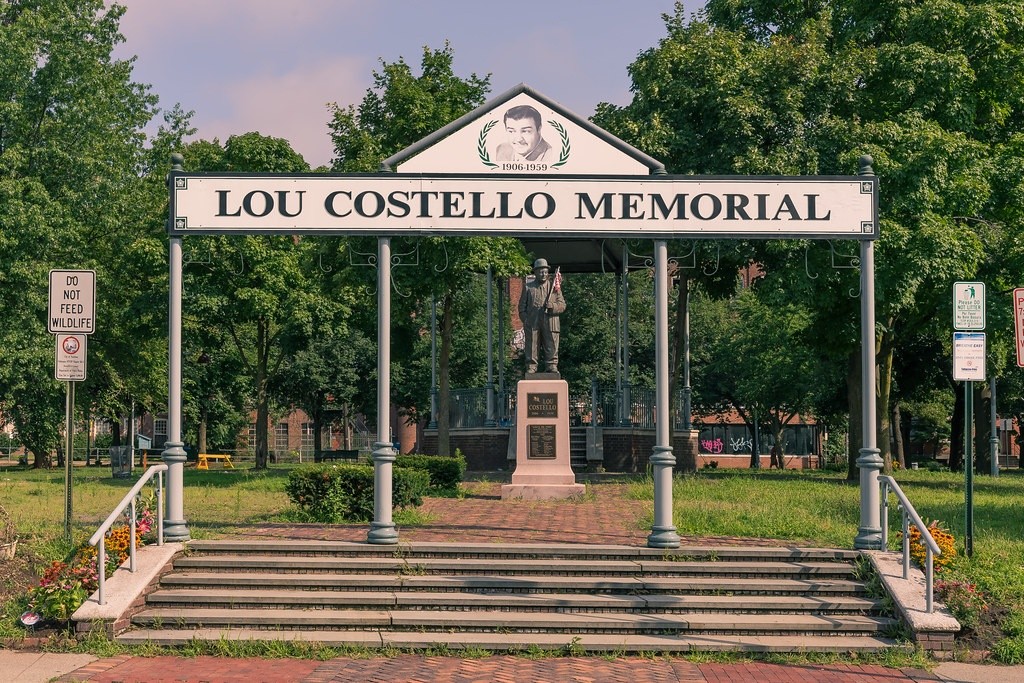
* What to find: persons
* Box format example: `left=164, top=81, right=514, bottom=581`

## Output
left=518, top=258, right=566, bottom=373
left=496, top=105, right=553, bottom=163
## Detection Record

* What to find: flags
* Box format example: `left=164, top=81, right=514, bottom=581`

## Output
left=554, top=272, right=563, bottom=292
